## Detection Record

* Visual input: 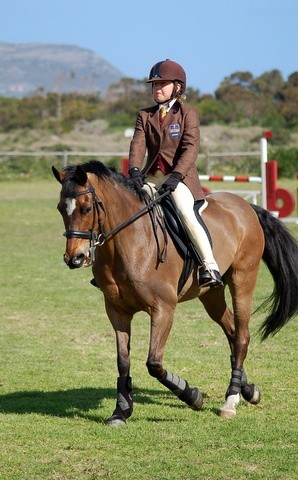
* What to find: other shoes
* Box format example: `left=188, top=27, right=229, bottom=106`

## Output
left=201, top=271, right=223, bottom=287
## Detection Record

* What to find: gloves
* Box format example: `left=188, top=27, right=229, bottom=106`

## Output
left=162, top=172, right=180, bottom=192
left=130, top=170, right=144, bottom=191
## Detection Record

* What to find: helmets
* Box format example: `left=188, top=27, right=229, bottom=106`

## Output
left=146, top=59, right=187, bottom=83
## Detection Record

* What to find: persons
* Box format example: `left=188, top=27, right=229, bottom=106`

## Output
left=89, top=59, right=226, bottom=291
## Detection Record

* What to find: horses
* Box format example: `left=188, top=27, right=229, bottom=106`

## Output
left=51, top=160, right=298, bottom=427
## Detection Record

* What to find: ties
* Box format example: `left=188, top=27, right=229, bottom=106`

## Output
left=160, top=107, right=166, bottom=118
